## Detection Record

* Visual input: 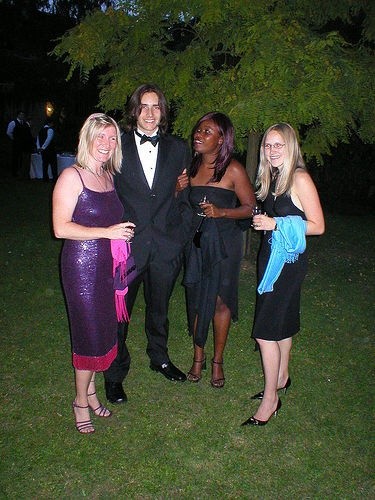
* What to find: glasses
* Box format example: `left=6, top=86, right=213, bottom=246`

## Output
left=264, top=143, right=286, bottom=149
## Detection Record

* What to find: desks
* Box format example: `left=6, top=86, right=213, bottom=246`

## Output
left=29, top=152, right=76, bottom=181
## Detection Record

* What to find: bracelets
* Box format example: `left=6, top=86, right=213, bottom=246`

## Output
left=273, top=223, right=277, bottom=231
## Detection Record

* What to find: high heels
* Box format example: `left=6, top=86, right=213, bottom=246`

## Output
left=210, top=358, right=226, bottom=388
left=240, top=398, right=282, bottom=427
left=72, top=399, right=95, bottom=434
left=87, top=391, right=112, bottom=417
left=249, top=376, right=292, bottom=400
left=187, top=356, right=207, bottom=382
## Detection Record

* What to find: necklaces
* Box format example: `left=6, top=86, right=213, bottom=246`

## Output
left=86, top=165, right=108, bottom=193
left=272, top=170, right=284, bottom=201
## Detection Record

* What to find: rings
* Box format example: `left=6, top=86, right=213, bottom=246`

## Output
left=125, top=229, right=127, bottom=231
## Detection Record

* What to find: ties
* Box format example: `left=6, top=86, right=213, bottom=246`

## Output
left=20, top=121, right=23, bottom=126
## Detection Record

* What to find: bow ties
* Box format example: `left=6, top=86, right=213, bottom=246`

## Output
left=134, top=128, right=159, bottom=147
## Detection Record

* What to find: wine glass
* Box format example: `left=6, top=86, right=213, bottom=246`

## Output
left=124, top=221, right=135, bottom=243
left=251, top=204, right=261, bottom=227
left=197, top=193, right=209, bottom=217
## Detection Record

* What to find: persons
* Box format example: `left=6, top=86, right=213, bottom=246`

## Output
left=36, top=119, right=58, bottom=183
left=7, top=111, right=26, bottom=178
left=164, top=111, right=257, bottom=388
left=19, top=116, right=40, bottom=183
left=240, top=121, right=324, bottom=427
left=101, top=84, right=192, bottom=406
left=52, top=112, right=136, bottom=435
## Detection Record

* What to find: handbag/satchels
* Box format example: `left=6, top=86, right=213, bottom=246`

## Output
left=113, top=240, right=138, bottom=290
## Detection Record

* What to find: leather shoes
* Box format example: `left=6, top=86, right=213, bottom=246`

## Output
left=104, top=381, right=128, bottom=405
left=149, top=354, right=187, bottom=382
